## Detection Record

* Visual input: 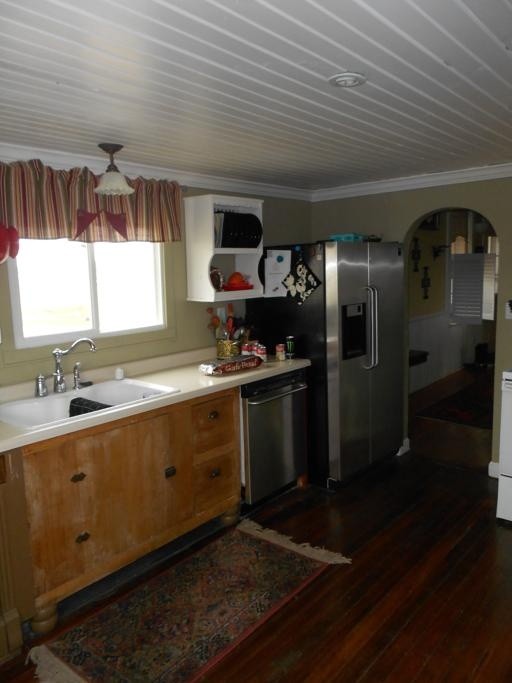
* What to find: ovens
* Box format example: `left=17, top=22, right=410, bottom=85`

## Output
left=242, top=367, right=309, bottom=505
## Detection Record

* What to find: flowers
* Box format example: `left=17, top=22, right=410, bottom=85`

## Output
left=207, top=303, right=251, bottom=340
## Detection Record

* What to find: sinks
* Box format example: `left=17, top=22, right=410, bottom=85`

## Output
left=0, top=378, right=111, bottom=450
left=71, top=378, right=180, bottom=408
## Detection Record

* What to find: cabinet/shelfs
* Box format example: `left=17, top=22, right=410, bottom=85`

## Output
left=184, top=386, right=241, bottom=535
left=23, top=402, right=184, bottom=610
left=184, top=195, right=264, bottom=303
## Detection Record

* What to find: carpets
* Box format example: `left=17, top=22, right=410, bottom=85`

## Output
left=25, top=518, right=352, bottom=683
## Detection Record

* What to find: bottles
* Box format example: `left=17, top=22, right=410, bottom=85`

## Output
left=34, top=375, right=49, bottom=397
left=241, top=343, right=268, bottom=362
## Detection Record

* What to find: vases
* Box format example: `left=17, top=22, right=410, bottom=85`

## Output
left=217, top=340, right=241, bottom=360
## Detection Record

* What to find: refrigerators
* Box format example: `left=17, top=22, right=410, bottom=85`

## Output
left=246, top=240, right=410, bottom=486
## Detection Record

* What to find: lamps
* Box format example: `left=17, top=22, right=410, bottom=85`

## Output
left=94, top=143, right=135, bottom=196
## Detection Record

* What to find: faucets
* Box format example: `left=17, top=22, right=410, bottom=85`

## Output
left=52, top=337, right=97, bottom=393
